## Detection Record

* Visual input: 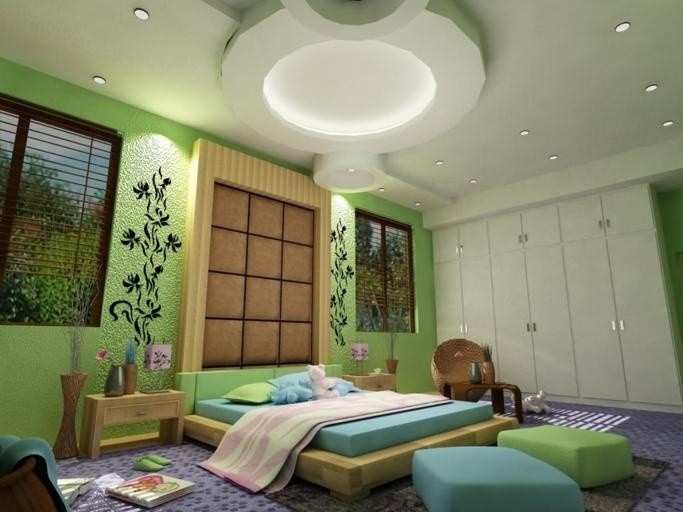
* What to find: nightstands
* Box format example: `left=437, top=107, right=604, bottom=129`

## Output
left=79, top=388, right=187, bottom=458
left=342, top=373, right=398, bottom=391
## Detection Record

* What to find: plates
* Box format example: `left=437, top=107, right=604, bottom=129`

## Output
left=368, top=372, right=385, bottom=375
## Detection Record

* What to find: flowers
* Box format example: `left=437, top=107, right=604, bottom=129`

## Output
left=95, top=349, right=120, bottom=367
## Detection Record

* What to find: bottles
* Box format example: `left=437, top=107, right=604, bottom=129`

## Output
left=468, top=361, right=481, bottom=383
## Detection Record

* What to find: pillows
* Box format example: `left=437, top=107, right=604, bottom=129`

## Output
left=268, top=372, right=310, bottom=389
left=221, top=382, right=278, bottom=404
left=329, top=378, right=360, bottom=396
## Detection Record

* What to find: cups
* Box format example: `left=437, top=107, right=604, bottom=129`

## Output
left=373, top=367, right=382, bottom=373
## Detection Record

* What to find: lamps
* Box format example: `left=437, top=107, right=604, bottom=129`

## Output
left=351, top=341, right=370, bottom=376
left=139, top=343, right=172, bottom=393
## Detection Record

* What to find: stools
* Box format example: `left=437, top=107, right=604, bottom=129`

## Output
left=407, top=444, right=583, bottom=512
left=497, top=423, right=635, bottom=487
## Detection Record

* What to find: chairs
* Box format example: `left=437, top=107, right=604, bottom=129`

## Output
left=430, top=338, right=494, bottom=402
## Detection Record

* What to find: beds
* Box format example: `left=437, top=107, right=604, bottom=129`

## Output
left=176, top=364, right=518, bottom=501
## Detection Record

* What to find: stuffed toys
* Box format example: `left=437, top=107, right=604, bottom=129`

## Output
left=269, top=363, right=340, bottom=404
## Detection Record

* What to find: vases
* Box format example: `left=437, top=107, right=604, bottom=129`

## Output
left=0, top=454, right=67, bottom=512
left=469, top=361, right=481, bottom=384
left=53, top=370, right=89, bottom=460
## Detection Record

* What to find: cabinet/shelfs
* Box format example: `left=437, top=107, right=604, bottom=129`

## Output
left=562, top=183, right=683, bottom=413
left=431, top=217, right=498, bottom=382
left=487, top=200, right=578, bottom=405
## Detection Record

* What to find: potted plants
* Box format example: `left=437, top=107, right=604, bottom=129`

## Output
left=385, top=313, right=399, bottom=372
left=120, top=342, right=137, bottom=394
left=480, top=345, right=495, bottom=385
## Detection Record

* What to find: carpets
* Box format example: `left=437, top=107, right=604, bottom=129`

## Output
left=264, top=438, right=670, bottom=512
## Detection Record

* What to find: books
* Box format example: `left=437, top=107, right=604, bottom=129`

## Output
left=107, top=472, right=195, bottom=508
left=56, top=478, right=91, bottom=506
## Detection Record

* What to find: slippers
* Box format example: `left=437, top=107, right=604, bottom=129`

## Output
left=132, top=454, right=173, bottom=473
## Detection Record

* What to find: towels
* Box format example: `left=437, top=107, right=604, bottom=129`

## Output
left=0, top=434, right=72, bottom=512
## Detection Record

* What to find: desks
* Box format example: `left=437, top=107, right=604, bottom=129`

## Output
left=449, top=381, right=525, bottom=424
